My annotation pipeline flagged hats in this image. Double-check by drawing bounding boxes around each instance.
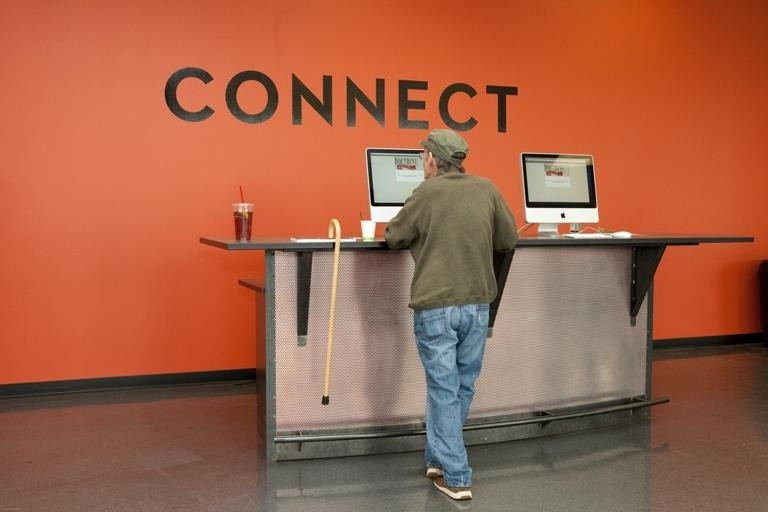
[418,128,470,165]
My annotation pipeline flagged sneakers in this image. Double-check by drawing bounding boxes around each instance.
[427,466,473,501]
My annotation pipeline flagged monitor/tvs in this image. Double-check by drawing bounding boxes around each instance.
[521,153,599,236]
[365,147,424,223]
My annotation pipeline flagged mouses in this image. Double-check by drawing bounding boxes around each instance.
[612,231,632,239]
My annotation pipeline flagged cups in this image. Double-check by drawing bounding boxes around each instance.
[359,219,376,242]
[231,202,255,242]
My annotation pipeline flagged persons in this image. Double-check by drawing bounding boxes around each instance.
[384,131,518,501]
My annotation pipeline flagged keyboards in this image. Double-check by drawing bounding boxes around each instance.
[563,233,613,239]
